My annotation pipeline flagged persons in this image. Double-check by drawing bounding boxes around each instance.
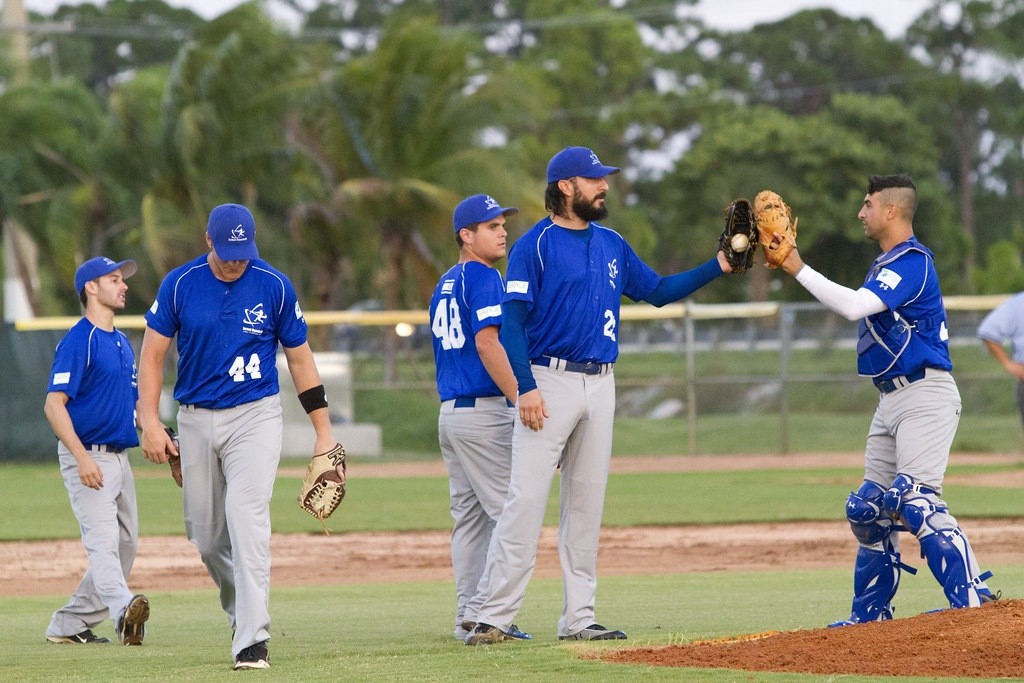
[755,174,993,628]
[428,194,531,642]
[45,257,150,646]
[138,204,347,671]
[466,146,758,646]
[977,293,1024,423]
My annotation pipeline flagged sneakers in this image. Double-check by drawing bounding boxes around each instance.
[115,594,152,647]
[464,622,505,646]
[828,616,886,627]
[462,619,531,640]
[47,629,112,644]
[558,625,628,641]
[234,643,270,670]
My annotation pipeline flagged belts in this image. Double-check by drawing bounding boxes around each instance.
[875,369,926,395]
[454,395,515,408]
[531,356,614,376]
[186,403,200,409]
[82,442,126,453]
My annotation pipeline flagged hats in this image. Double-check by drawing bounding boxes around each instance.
[207,203,260,261]
[547,146,622,185]
[75,256,137,297]
[452,193,518,233]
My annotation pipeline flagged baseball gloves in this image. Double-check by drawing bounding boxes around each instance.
[297,442,348,538]
[165,425,183,488]
[717,197,760,275]
[754,190,799,271]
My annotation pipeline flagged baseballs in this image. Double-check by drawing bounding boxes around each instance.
[730,233,750,253]
